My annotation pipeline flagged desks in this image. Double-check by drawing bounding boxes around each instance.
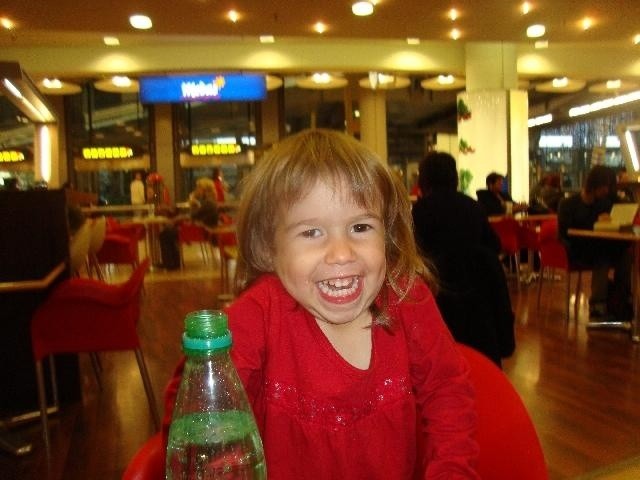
[567,226,640,342]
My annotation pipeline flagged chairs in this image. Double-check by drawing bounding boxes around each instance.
[489,215,584,305]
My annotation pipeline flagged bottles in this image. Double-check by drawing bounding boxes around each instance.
[165,309,269,480]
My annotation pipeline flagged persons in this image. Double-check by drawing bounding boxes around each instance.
[476,172,542,274]
[412,150,517,372]
[556,163,636,323]
[151,177,220,272]
[212,164,235,227]
[129,169,147,215]
[120,128,549,479]
